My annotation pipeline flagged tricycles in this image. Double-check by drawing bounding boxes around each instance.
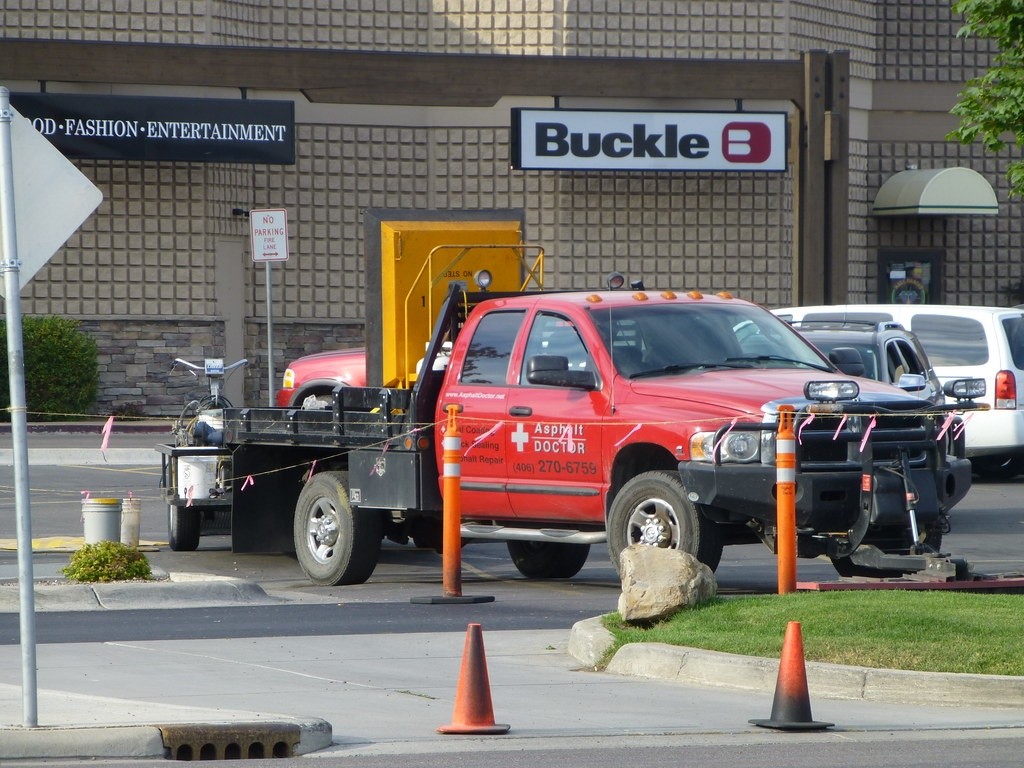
[155,356,250,551]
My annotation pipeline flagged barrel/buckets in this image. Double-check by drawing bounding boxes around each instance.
[80,499,123,544]
[175,447,218,499]
[121,499,141,547]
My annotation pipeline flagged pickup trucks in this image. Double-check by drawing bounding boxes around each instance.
[218,270,972,588]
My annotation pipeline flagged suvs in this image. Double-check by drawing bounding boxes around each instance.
[731,304,1024,481]
[731,318,948,411]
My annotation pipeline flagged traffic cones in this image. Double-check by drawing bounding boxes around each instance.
[747,619,837,732]
[435,624,514,735]
